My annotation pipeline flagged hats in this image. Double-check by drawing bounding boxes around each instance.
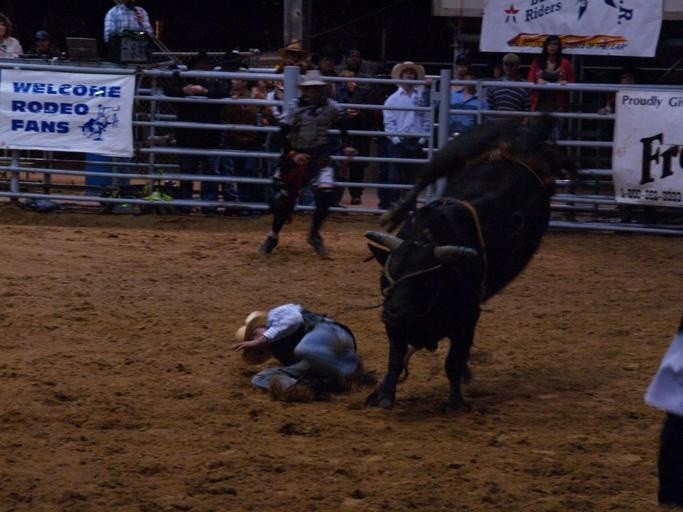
[234,310,273,366]
[34,30,53,40]
[454,53,472,64]
[216,49,250,69]
[295,68,330,89]
[278,38,313,62]
[189,52,218,69]
[502,53,521,66]
[311,47,344,65]
[392,59,426,88]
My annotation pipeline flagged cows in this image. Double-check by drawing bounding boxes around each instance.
[360,109,561,416]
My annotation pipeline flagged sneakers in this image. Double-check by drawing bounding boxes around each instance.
[202,206,223,216]
[223,207,271,217]
[350,195,362,205]
[306,231,329,259]
[256,231,279,254]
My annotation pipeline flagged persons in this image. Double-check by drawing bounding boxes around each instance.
[644,318,682,510]
[0,3,646,254]
[235,303,366,400]
